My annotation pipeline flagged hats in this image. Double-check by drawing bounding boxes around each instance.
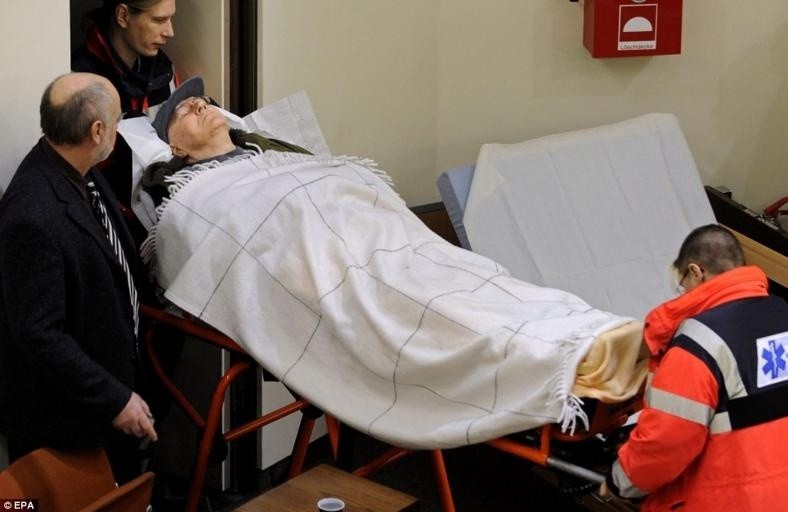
[152,74,205,144]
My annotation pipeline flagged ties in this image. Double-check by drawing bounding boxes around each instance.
[87,180,143,354]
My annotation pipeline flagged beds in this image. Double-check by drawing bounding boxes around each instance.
[105,122,651,504]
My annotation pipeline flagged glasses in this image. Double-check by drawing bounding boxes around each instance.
[170,94,212,125]
[675,268,706,296]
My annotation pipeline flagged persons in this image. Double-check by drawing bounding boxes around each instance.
[69,0,181,113]
[142,73,314,221]
[599,224,788,511]
[0,71,157,484]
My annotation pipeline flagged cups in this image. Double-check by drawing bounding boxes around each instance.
[316,496,346,512]
